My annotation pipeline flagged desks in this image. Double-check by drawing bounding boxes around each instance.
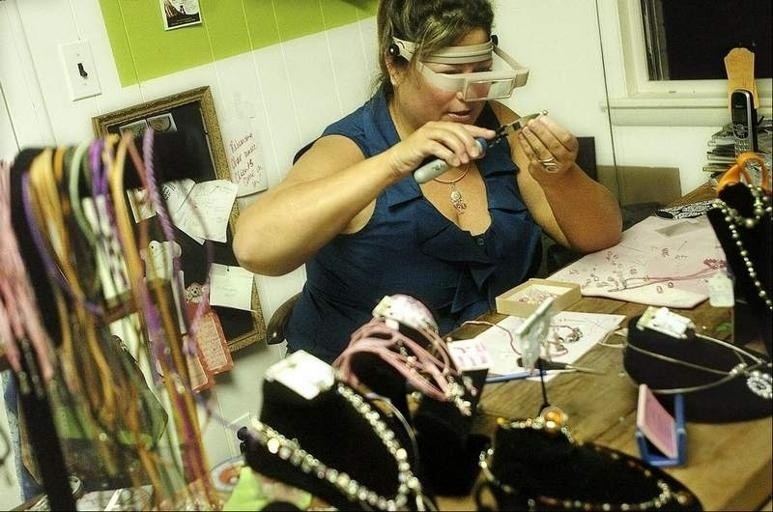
[141,186,773,512]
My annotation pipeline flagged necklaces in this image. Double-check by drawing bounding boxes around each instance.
[1,129,220,512]
[461,320,580,361]
[624,257,729,289]
[246,388,425,512]
[713,187,773,315]
[332,294,478,419]
[474,453,693,511]
[622,333,772,400]
[433,162,471,214]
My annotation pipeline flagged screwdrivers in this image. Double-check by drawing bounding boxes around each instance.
[518,357,608,376]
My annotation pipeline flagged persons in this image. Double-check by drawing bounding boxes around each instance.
[232,0,623,370]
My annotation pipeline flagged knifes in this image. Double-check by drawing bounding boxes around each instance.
[413,113,540,183]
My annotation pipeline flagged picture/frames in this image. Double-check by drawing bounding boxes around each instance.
[92,86,267,356]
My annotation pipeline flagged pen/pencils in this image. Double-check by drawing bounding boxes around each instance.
[485,370,546,383]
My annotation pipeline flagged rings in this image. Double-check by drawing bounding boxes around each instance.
[538,157,560,174]
